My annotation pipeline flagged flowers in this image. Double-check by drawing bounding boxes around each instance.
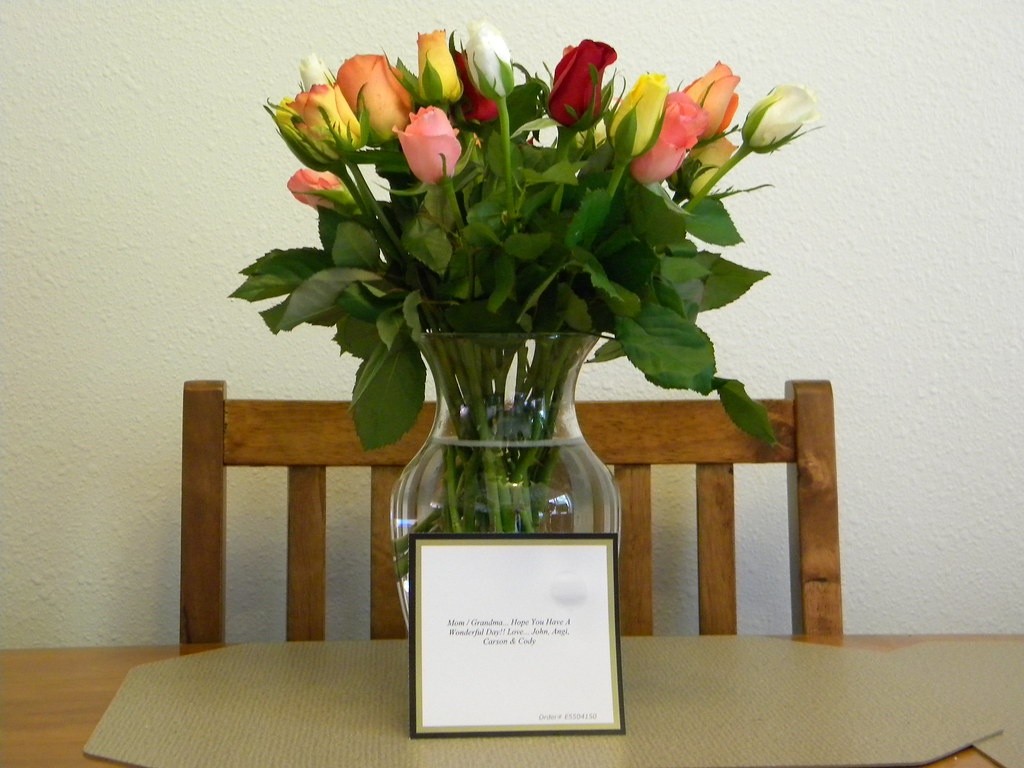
[229,26,825,452]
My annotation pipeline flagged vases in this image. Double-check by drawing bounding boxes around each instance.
[389,328,622,634]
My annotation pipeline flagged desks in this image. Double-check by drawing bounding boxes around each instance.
[0,635,1024,768]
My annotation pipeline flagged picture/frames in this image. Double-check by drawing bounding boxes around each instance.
[408,534,626,737]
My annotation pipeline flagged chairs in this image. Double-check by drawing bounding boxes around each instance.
[180,380,844,644]
